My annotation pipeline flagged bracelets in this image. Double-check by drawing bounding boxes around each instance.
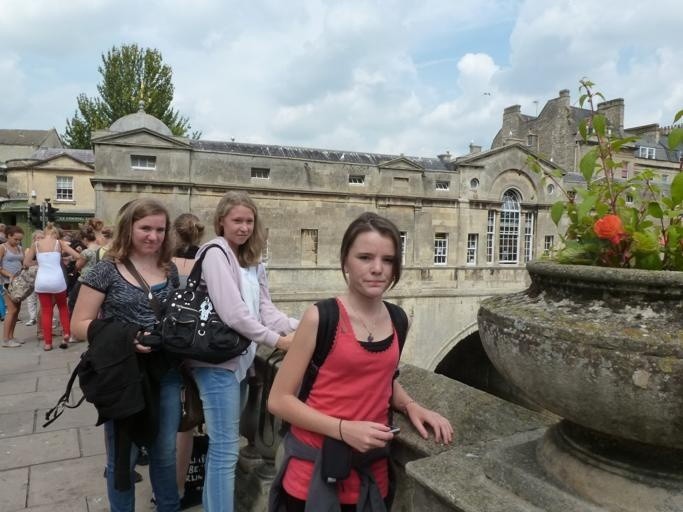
[401,398,414,416]
[339,418,344,442]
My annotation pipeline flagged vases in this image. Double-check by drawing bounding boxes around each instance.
[478,259,683,512]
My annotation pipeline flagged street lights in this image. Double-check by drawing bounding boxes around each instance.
[40,197,49,231]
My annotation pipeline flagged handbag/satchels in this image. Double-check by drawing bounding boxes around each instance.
[0,265,37,306]
[151,435,213,507]
[159,286,256,365]
[177,368,206,433]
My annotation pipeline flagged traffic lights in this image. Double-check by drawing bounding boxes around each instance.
[49,206,60,223]
[28,204,40,226]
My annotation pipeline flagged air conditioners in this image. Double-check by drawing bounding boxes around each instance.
[634,146,656,160]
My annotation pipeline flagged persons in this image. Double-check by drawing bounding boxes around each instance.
[150,212,205,509]
[69,197,181,512]
[186,191,301,512]
[104,449,150,482]
[265,213,454,512]
[0,217,116,351]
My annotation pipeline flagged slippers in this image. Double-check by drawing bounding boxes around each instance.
[58,336,70,350]
[42,344,53,352]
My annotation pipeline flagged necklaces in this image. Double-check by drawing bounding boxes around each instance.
[351,304,382,342]
[129,256,153,300]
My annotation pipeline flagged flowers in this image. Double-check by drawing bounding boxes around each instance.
[519,76,683,272]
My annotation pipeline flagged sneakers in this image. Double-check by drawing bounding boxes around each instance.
[0,338,25,349]
[23,317,36,327]
[149,483,203,510]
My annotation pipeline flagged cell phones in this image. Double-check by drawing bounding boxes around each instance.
[385,424,401,434]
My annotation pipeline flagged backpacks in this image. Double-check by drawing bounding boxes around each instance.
[275,292,405,437]
